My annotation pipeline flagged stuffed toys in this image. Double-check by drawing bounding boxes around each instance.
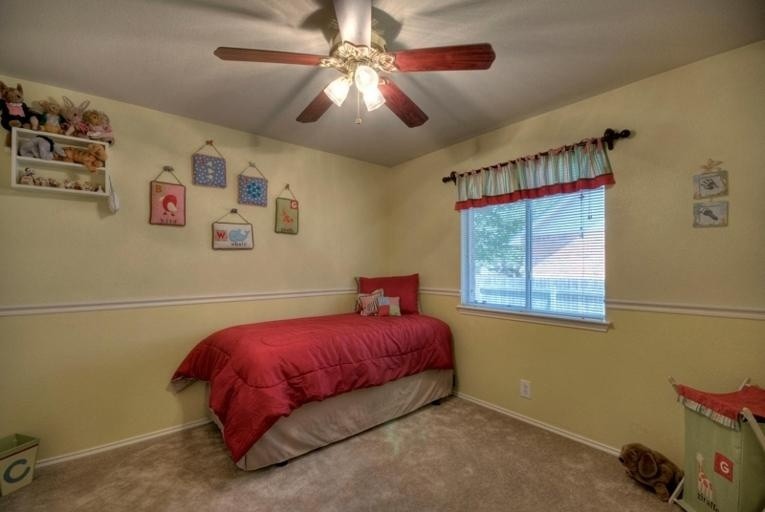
[0,81,41,130]
[19,135,66,161]
[619,443,684,501]
[84,111,114,145]
[37,100,65,134]
[52,144,106,174]
[62,96,91,137]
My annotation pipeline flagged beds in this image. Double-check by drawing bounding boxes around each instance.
[171,315,454,471]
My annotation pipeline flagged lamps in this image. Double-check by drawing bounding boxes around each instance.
[319,1,398,125]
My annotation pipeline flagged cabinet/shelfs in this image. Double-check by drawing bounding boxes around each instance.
[11,126,110,199]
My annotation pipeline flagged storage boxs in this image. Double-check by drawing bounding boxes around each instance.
[669,377,765,512]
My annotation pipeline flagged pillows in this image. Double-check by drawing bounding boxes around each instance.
[359,292,382,316]
[374,297,402,316]
[357,288,385,309]
[354,272,422,315]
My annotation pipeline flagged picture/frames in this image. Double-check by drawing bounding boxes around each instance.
[694,170,729,200]
[693,201,729,228]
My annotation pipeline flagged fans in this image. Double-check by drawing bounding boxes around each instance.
[213,1,496,129]
